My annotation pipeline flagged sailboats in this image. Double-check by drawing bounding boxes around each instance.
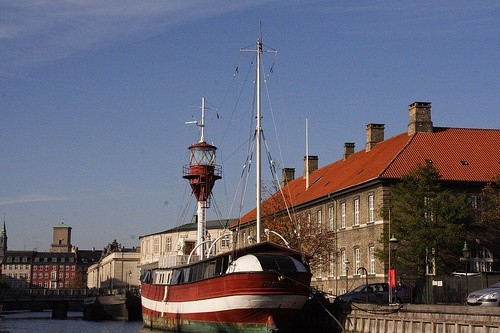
[139,17,314,333]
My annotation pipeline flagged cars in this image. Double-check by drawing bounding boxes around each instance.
[334,283,411,313]
[467,282,500,306]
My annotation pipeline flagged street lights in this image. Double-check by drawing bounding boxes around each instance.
[344,256,350,300]
[476,237,489,289]
[128,270,132,291]
[461,240,470,303]
[388,233,400,303]
